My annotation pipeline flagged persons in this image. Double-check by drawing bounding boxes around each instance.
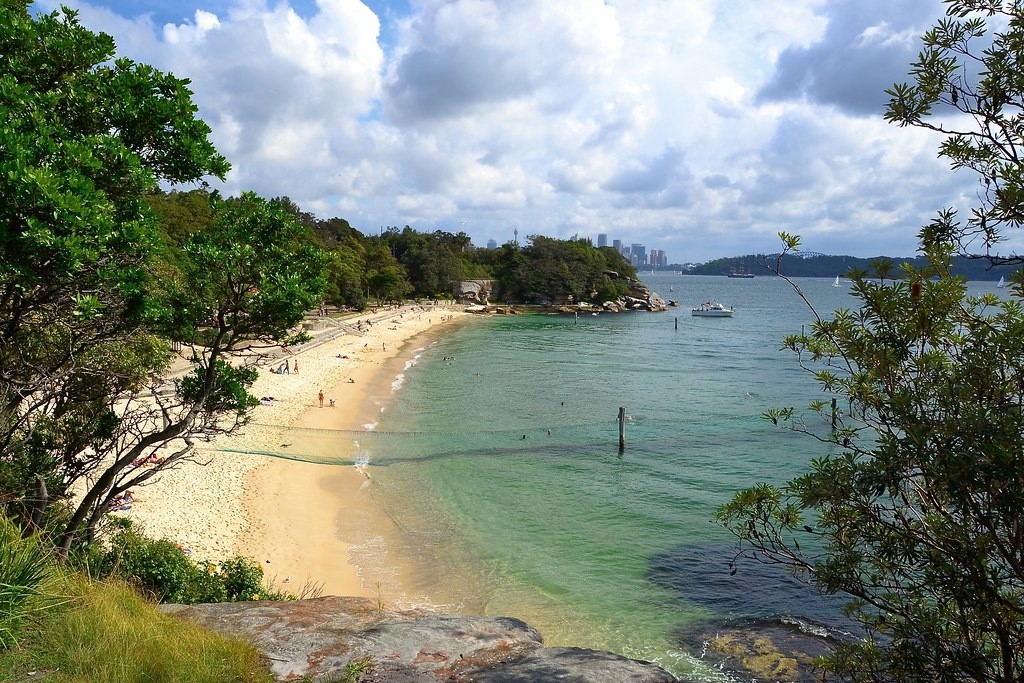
[132,452,168,468]
[319,389,338,407]
[318,303,453,331]
[477,373,479,376]
[282,347,299,375]
[522,401,564,439]
[443,356,455,361]
[338,342,385,358]
[109,490,139,507]
[348,377,355,383]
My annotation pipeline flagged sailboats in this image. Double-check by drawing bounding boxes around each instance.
[728,254,756,278]
[831,274,841,288]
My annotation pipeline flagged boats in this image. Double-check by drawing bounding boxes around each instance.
[692,301,734,317]
